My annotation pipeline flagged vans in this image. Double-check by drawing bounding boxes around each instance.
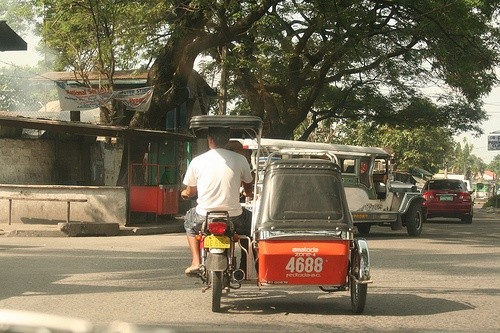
[227,138,424,236]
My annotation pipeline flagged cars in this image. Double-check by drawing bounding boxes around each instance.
[420,178,475,225]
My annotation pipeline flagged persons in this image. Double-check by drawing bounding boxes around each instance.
[181,128,253,275]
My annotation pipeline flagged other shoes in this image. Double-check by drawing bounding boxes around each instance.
[185,265,200,273]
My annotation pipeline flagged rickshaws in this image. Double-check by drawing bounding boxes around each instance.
[182,115,374,313]
